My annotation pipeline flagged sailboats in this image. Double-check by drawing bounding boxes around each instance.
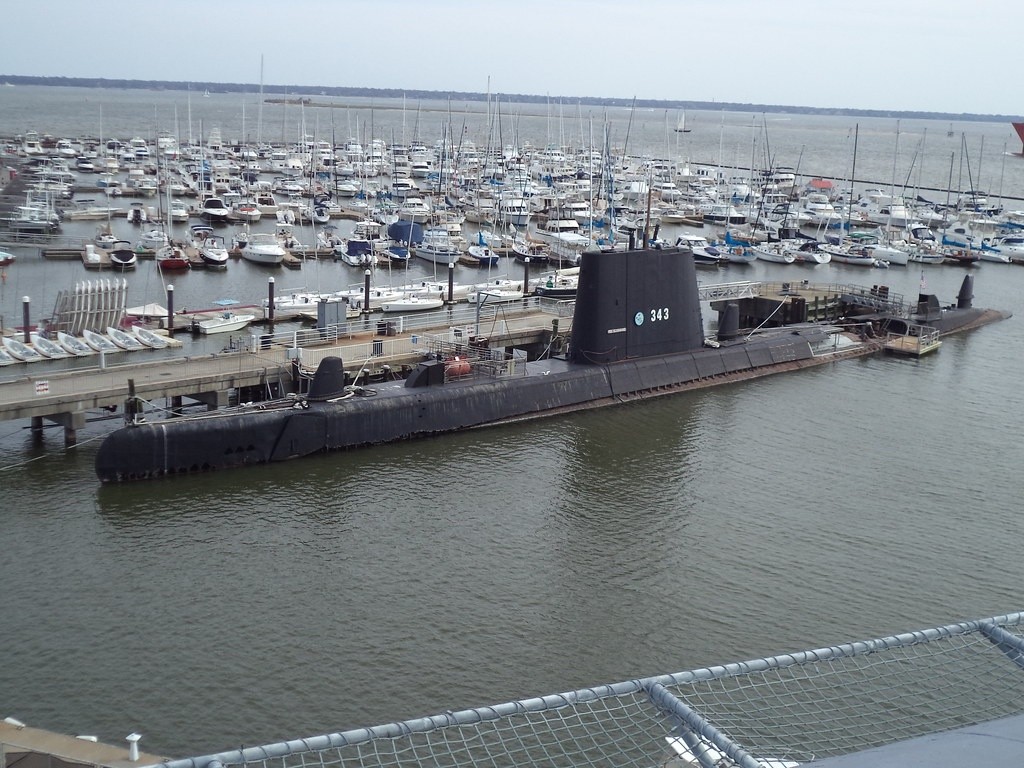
[0,74,1024,324]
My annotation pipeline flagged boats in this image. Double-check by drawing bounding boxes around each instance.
[0,349,14,366]
[30,334,68,359]
[83,330,118,354]
[108,327,142,350]
[187,306,256,335]
[58,332,93,356]
[132,325,168,349]
[1,336,42,363]
